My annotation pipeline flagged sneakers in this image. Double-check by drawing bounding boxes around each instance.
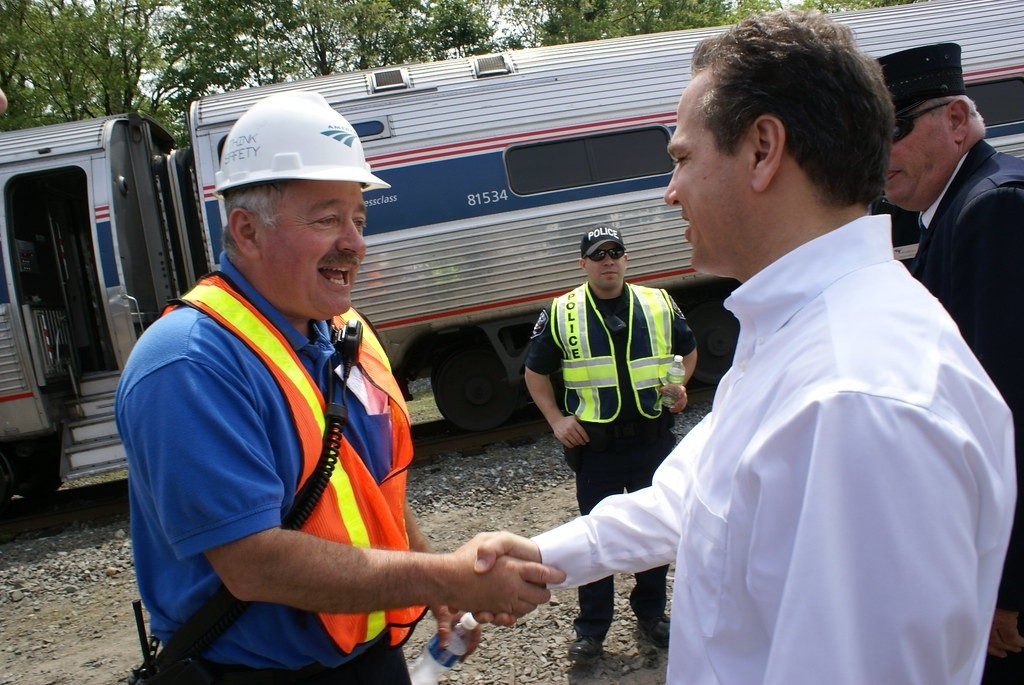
[636,614,670,647]
[568,635,604,665]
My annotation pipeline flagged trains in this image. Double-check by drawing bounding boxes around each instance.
[0,1,1024,524]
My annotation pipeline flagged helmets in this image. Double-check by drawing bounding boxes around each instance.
[214,93,393,192]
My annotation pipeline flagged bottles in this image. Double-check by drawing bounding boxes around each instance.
[664,355,685,407]
[409,612,479,685]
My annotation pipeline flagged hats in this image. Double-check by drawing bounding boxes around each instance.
[873,43,967,116]
[581,224,626,259]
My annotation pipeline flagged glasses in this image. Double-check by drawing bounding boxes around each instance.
[891,100,950,144]
[588,246,624,261]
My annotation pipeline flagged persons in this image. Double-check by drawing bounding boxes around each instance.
[876,41,1024,685]
[521,223,699,665]
[448,8,1017,685]
[114,92,566,685]
